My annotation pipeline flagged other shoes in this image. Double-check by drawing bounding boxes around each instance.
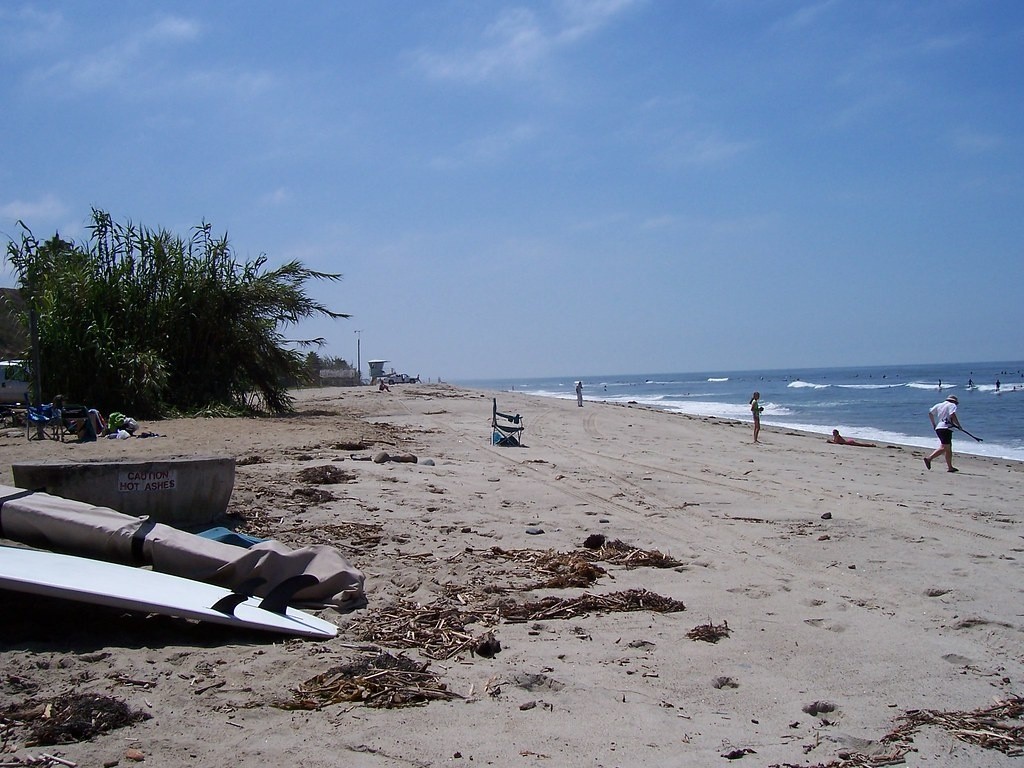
[947,467,958,472]
[924,458,932,470]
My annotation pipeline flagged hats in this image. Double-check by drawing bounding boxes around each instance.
[945,395,958,404]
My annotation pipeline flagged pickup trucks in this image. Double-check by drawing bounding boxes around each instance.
[383,373,416,385]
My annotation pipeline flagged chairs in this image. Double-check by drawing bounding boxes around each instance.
[492,398,524,448]
[24,391,63,441]
[49,406,97,442]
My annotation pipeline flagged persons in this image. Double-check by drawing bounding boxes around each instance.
[939,379,941,386]
[750,392,764,443]
[968,379,973,388]
[923,395,962,472]
[576,382,583,407]
[379,380,391,392]
[826,430,876,447]
[995,379,1000,392]
[51,395,75,433]
[417,375,421,383]
